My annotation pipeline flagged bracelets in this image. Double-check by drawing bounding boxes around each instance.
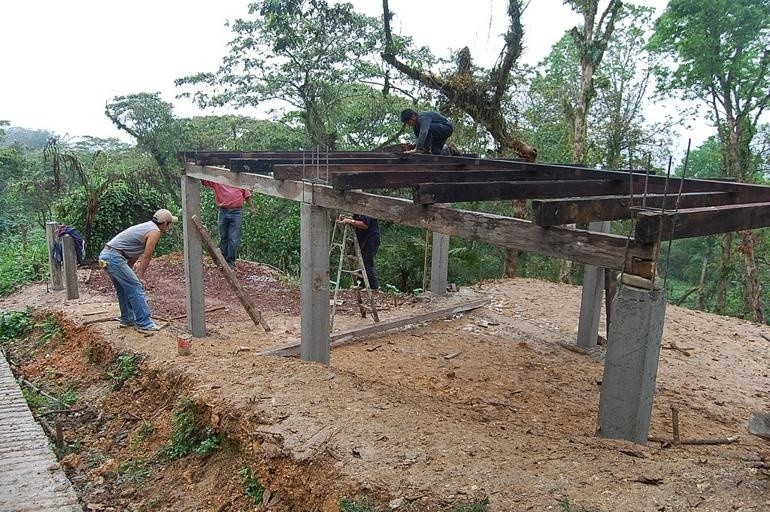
[351,219,356,226]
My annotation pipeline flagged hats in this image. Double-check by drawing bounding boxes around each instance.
[153,209,179,224]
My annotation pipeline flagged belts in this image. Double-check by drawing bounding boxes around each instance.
[104,244,126,256]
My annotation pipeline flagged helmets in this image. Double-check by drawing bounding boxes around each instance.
[401,109,413,122]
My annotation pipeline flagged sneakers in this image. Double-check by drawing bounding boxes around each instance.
[120,322,134,328]
[134,325,161,331]
[449,142,463,157]
[353,286,379,292]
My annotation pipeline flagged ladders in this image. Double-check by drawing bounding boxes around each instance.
[191,213,272,332]
[329,215,380,333]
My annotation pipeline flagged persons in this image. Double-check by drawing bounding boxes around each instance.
[399,109,465,156]
[335,187,382,292]
[97,207,180,332]
[201,180,257,265]
